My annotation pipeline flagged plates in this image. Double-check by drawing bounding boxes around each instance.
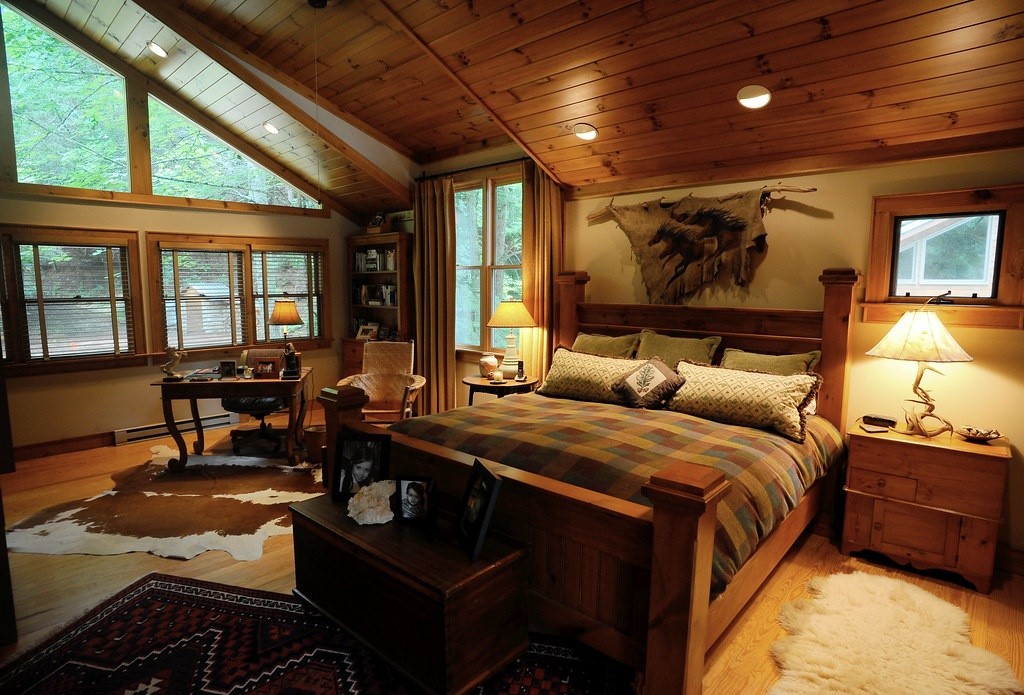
[490,380,507,383]
[955,431,1002,441]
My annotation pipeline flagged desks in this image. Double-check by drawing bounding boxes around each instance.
[461,374,539,407]
[149,366,314,466]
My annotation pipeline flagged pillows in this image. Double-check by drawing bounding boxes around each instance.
[610,355,686,411]
[634,329,722,371]
[719,347,822,377]
[569,331,641,358]
[534,343,650,404]
[664,358,824,443]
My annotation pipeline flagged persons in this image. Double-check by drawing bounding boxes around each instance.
[339,447,373,492]
[401,482,426,518]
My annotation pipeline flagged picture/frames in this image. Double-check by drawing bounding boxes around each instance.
[450,457,502,564]
[394,475,435,523]
[355,325,380,339]
[331,431,390,505]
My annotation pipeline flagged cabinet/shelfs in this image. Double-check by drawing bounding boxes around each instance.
[344,232,412,375]
[840,419,1013,594]
[287,494,530,695]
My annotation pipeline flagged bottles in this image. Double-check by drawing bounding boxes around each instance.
[479,352,500,376]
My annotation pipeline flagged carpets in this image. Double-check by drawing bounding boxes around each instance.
[4,421,331,561]
[0,573,646,694]
[767,570,1024,695]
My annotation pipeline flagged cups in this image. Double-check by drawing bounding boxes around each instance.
[493,370,504,381]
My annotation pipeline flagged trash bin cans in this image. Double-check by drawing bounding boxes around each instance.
[303,425,327,463]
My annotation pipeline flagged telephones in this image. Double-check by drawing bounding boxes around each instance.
[515,361,527,382]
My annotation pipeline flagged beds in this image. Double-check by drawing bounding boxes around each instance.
[314,267,864,695]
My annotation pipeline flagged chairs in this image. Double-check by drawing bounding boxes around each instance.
[220,349,305,453]
[337,339,427,430]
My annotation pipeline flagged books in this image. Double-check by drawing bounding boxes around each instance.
[352,316,370,336]
[351,280,396,308]
[355,248,397,271]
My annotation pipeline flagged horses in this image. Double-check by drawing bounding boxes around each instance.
[160,347,188,376]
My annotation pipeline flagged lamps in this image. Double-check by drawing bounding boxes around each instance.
[267,300,305,372]
[865,300,974,437]
[486,301,539,378]
